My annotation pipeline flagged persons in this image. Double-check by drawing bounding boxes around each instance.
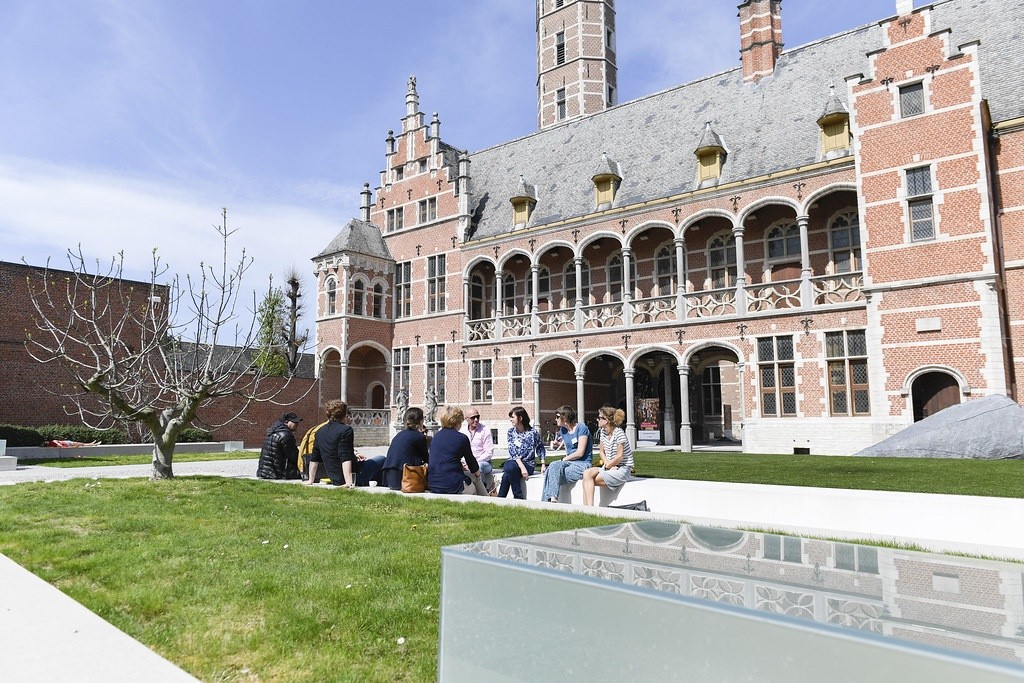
[297,400,385,488]
[640,417,659,430]
[497,406,546,500]
[547,431,564,451]
[41,440,101,446]
[428,405,496,496]
[420,425,432,447]
[540,405,593,503]
[381,407,429,490]
[256,412,302,479]
[582,406,635,505]
[587,419,596,436]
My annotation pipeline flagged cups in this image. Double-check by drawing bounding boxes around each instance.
[349,473,356,489]
[369,481,378,487]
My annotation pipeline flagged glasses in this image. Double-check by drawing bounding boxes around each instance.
[468,415,480,420]
[597,416,607,420]
[556,415,561,419]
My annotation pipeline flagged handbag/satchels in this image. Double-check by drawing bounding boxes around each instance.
[401,463,428,493]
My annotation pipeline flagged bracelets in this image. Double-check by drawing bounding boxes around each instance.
[541,463,545,464]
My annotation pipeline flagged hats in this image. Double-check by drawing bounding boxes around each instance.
[280,412,304,422]
